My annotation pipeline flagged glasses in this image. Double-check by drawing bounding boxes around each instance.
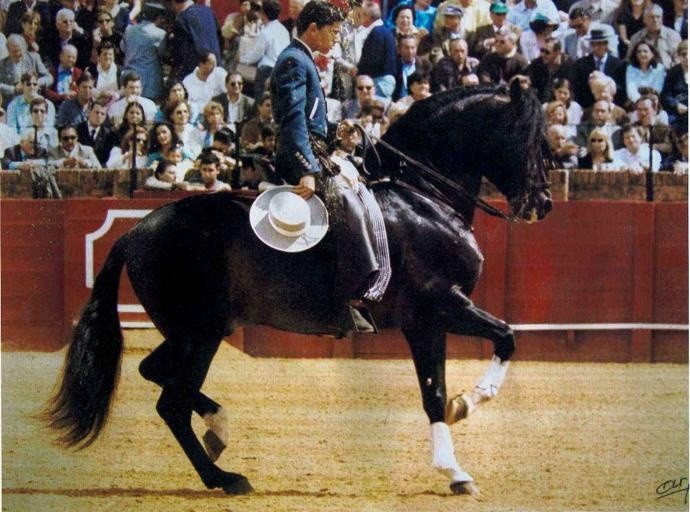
[230,80,243,88]
[539,47,552,56]
[358,85,374,92]
[61,135,78,143]
[591,138,606,143]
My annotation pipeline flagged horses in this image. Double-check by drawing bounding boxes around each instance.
[20,74,556,497]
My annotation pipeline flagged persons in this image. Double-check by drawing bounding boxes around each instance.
[0,0,688,193]
[271,0,394,339]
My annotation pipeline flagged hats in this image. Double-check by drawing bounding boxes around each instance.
[583,25,619,44]
[248,182,329,253]
[441,5,464,18]
[489,1,509,15]
